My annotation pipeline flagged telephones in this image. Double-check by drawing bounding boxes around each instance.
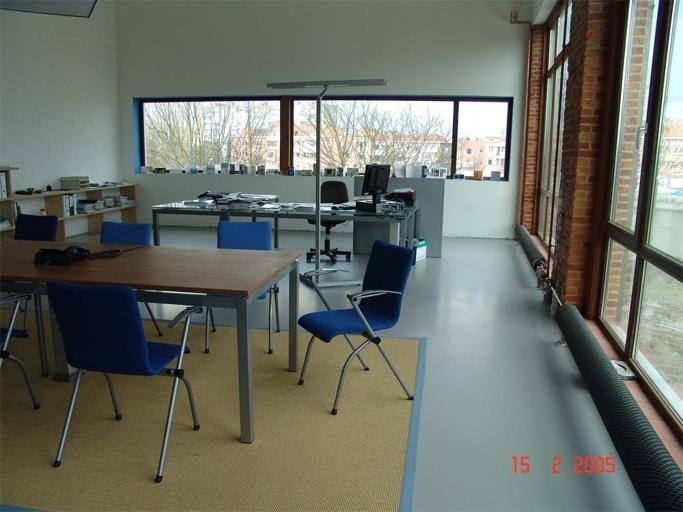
[35,245,122,265]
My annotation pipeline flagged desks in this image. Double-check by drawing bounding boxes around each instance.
[0,239,304,445]
[152,198,419,249]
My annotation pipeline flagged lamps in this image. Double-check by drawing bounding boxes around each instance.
[266,78,388,287]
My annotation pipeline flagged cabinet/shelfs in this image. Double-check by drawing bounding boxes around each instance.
[0,183,140,240]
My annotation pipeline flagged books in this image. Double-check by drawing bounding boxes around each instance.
[65,194,77,216]
[78,199,104,214]
[258,202,282,212]
[104,195,129,208]
[218,196,258,212]
[314,202,356,217]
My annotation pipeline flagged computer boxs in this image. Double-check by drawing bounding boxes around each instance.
[357,199,401,214]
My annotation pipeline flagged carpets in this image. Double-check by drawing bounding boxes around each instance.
[0,306,427,512]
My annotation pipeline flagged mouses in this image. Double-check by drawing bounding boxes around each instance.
[330,205,340,211]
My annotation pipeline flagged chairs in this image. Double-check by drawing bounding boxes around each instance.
[305,181,351,263]
[297,238,415,416]
[205,221,280,355]
[0,293,41,410]
[46,281,204,482]
[14,213,59,329]
[99,221,163,337]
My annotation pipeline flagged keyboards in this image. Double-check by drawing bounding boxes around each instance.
[339,205,356,210]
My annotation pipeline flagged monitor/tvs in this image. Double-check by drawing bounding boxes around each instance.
[360,163,388,205]
